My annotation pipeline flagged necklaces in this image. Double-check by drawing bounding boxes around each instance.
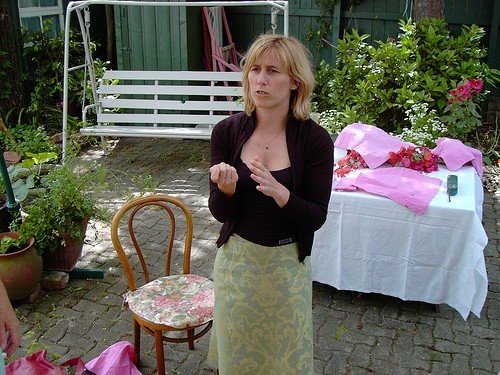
[260,127,285,149]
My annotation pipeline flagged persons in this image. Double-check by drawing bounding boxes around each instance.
[205,34,335,375]
[0,279,21,375]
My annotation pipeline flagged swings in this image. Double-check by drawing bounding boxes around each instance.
[78,5,279,139]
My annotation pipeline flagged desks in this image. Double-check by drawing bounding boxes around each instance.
[311,166,489,321]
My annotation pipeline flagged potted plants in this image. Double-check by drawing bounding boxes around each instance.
[0,122,166,306]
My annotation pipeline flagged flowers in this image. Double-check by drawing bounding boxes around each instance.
[387,103,449,172]
[334,147,368,176]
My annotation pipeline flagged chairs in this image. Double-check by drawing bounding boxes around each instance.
[110,194,221,375]
